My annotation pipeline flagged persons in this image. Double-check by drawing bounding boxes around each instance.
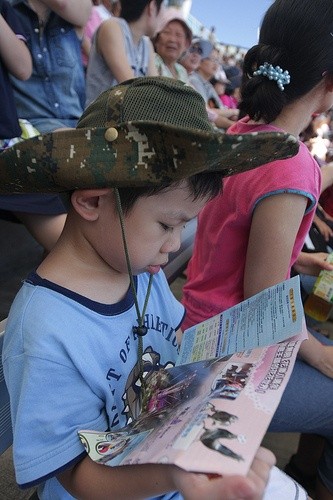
[175,0,333,435]
[0,76,312,500]
[0,0,333,322]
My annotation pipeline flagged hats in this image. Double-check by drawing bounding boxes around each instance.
[0,75,300,191]
[151,7,193,50]
[191,34,214,60]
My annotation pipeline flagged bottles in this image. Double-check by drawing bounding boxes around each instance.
[304,253,333,323]
[308,112,330,164]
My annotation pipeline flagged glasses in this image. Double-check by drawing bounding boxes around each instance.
[189,46,205,54]
